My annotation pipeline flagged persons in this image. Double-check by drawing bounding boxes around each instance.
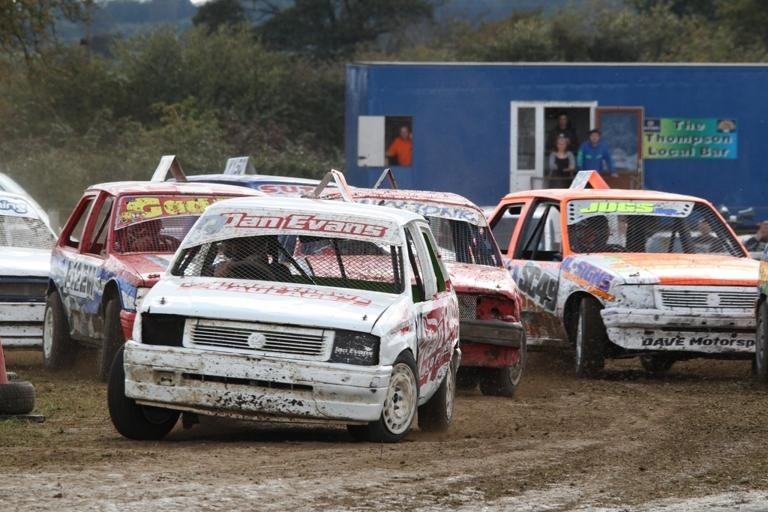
[547,132,575,175]
[386,121,413,166]
[743,217,767,252]
[694,213,726,253]
[574,128,620,178]
[546,112,576,154]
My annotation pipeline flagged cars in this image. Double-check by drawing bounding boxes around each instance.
[108,169,466,442]
[43,154,271,385]
[0,174,57,368]
[468,168,761,378]
[169,155,362,195]
[645,229,731,255]
[476,203,564,260]
[304,170,532,396]
[753,219,767,376]
